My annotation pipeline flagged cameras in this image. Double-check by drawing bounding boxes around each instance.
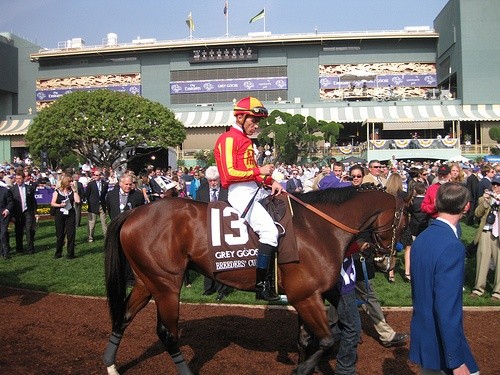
[347,176,353,181]
[489,191,496,198]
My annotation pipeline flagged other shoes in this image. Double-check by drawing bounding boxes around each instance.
[66,253,72,259]
[489,296,500,301]
[470,293,483,299]
[89,239,93,242]
[382,333,407,346]
[404,274,412,283]
[54,254,62,259]
[389,272,396,283]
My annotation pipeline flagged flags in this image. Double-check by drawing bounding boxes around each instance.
[224,2,227,15]
[249,9,264,24]
[186,15,194,30]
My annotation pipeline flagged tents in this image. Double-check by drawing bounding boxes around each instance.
[338,69,379,98]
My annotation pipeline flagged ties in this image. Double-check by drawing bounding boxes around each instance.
[18,187,24,209]
[492,196,500,238]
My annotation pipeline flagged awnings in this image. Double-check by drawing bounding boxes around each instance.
[0,105,500,135]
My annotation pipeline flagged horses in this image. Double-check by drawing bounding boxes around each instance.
[97,181,419,375]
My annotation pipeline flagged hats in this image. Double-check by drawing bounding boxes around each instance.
[438,164,451,175]
[490,174,500,183]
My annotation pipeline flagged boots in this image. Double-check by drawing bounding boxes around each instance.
[254,267,281,301]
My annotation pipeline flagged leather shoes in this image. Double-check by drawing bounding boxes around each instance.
[203,287,217,296]
[217,292,232,301]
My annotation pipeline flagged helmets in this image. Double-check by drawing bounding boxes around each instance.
[233,97,269,135]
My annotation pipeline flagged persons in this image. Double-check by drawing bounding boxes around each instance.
[324,140,360,148]
[347,82,457,100]
[370,131,376,140]
[421,160,500,300]
[436,131,453,138]
[409,182,480,375]
[214,96,282,302]
[465,139,471,145]
[275,160,429,375]
[0,155,228,300]
[257,143,274,165]
[413,132,420,139]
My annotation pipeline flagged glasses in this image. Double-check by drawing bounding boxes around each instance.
[233,106,269,115]
[93,174,98,176]
[370,166,381,169]
[491,182,500,185]
[351,175,362,178]
[293,173,298,175]
[422,172,428,176]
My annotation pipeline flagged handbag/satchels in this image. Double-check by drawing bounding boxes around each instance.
[49,206,56,216]
